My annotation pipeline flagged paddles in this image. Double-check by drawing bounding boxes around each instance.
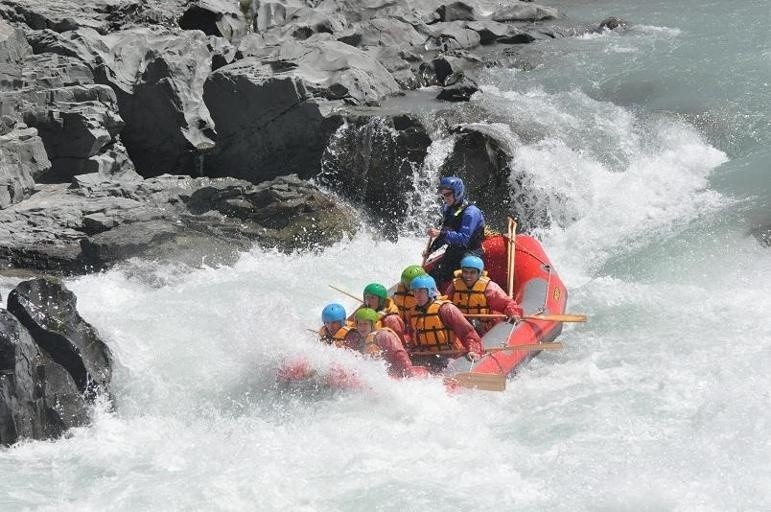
[444,372,506,392]
[463,313,587,323]
[410,342,564,356]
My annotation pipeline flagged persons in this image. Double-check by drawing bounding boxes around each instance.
[421,177,485,287]
[354,307,412,378]
[390,265,426,322]
[318,304,363,354]
[361,283,405,340]
[407,275,482,372]
[447,254,521,338]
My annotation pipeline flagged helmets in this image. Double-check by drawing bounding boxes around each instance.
[401,265,427,283]
[409,274,436,300]
[363,283,388,307]
[321,304,347,328]
[459,255,485,274]
[436,176,465,206]
[353,308,378,329]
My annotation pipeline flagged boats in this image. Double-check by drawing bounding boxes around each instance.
[277,233,569,398]
